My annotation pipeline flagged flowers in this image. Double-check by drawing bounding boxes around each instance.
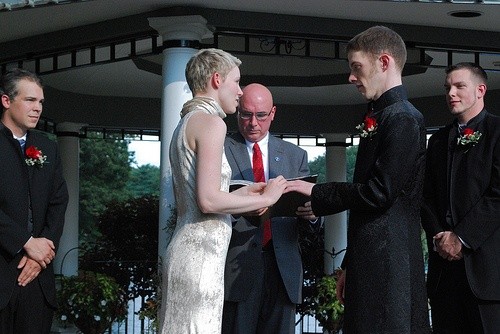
[55,270,129,334]
[354,117,379,142]
[456,127,482,151]
[23,145,52,170]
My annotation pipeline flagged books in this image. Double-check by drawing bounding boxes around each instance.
[230,173,318,217]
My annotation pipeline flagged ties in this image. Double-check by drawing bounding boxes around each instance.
[251,143,272,249]
[19,138,26,153]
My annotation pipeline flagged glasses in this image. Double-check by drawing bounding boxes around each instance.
[238,107,273,121]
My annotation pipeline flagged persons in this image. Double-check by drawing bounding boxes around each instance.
[0,67,69,334]
[283,25,434,334]
[224,82,324,334]
[420,62,500,334]
[158,48,287,334]
